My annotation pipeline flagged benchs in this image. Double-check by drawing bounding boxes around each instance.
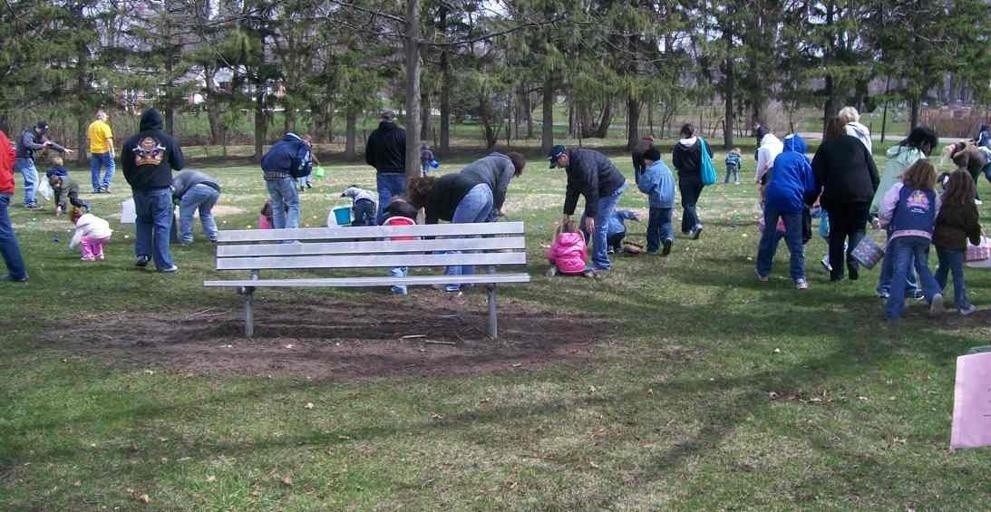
[203,221,530,340]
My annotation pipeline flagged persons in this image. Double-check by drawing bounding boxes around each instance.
[606,206,643,254]
[257,129,321,245]
[545,220,594,279]
[547,144,628,272]
[632,133,656,185]
[120,107,184,272]
[171,169,223,245]
[723,146,741,184]
[336,109,527,294]
[638,149,676,255]
[672,123,712,240]
[752,106,880,289]
[0,104,118,281]
[870,123,991,321]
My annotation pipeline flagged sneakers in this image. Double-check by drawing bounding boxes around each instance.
[3,201,177,282]
[755,258,975,317]
[545,223,703,279]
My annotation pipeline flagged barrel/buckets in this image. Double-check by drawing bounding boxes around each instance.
[332,198,351,225]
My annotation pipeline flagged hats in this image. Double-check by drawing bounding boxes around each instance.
[546,145,565,168]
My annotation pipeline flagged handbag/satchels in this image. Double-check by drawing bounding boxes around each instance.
[965,230,989,260]
[701,154,715,186]
